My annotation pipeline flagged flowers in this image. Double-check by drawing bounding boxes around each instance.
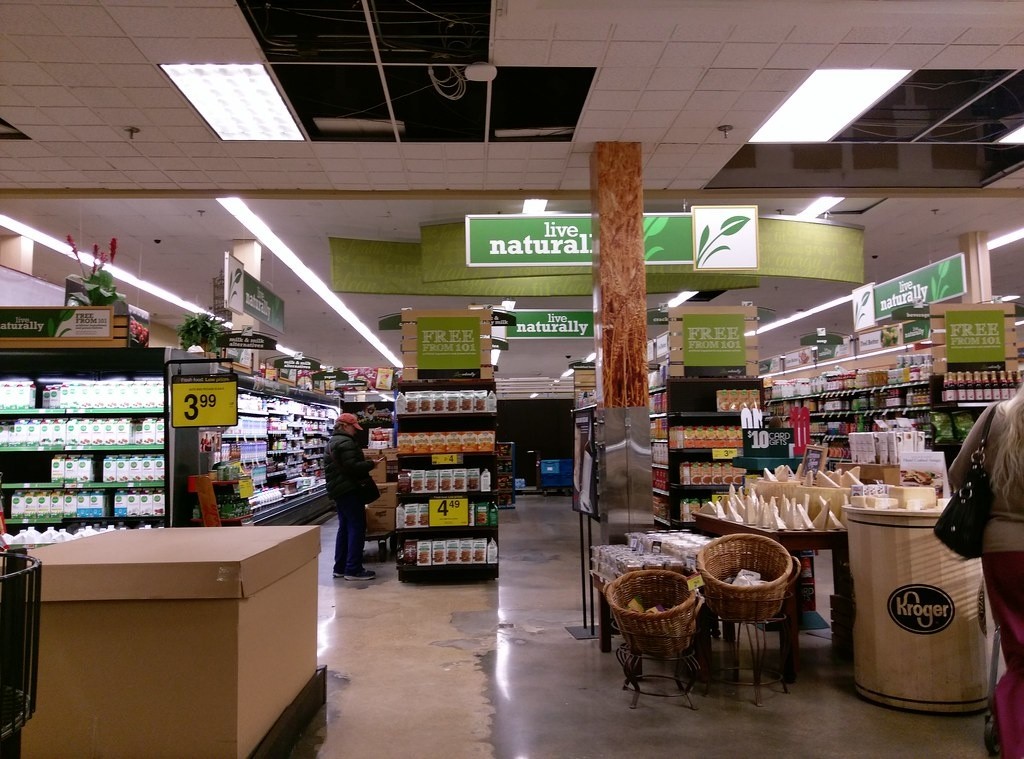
[65,232,127,308]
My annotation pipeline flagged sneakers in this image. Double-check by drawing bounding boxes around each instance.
[344,569,376,581]
[333,569,344,578]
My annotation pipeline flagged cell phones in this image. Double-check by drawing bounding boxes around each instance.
[379,457,385,460]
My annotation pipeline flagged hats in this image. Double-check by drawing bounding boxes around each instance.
[335,413,363,431]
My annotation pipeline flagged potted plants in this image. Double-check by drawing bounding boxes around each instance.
[178,310,220,353]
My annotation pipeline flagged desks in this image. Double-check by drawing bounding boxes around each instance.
[691,509,848,683]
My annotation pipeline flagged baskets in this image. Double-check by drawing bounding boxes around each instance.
[603,568,705,659]
[690,533,801,622]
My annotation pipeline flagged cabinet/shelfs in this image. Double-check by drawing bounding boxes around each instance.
[574,302,1024,526]
[393,379,500,582]
[496,441,516,509]
[188,473,254,527]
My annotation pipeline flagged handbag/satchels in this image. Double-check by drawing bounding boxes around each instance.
[933,402,998,559]
[348,473,381,505]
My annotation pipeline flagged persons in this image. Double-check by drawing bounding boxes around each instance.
[324,414,380,580]
[948,384,1024,759]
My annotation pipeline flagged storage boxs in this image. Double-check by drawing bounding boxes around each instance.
[0,525,321,759]
[742,428,798,458]
[361,430,496,534]
[376,367,393,391]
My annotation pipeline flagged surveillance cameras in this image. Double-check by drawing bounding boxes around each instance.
[566,355,571,358]
[872,255,878,259]
[154,239,161,243]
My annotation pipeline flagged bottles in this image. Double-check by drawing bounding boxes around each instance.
[11,521,166,545]
[480,468,492,491]
[766,360,1024,446]
